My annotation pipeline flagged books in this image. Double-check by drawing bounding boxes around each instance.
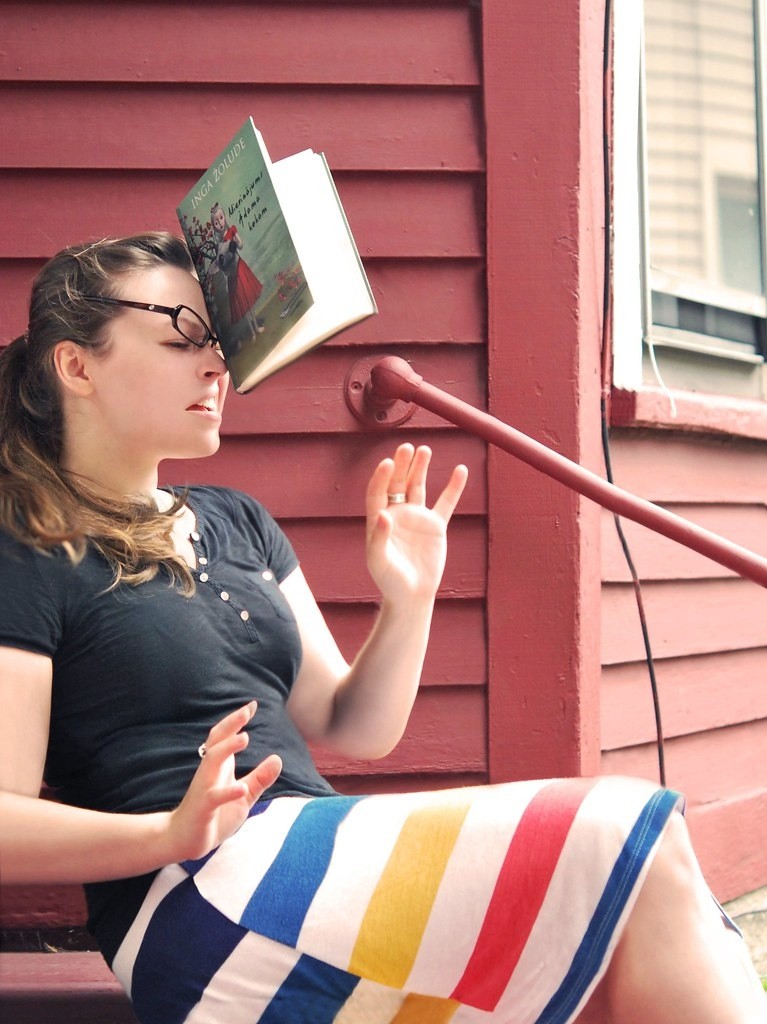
[177,114,379,396]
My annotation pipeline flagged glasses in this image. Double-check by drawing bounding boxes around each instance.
[81,295,220,348]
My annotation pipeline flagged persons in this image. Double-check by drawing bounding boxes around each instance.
[0,230,767,1024]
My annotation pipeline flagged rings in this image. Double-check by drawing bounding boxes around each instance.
[389,492,405,503]
[198,744,207,758]
